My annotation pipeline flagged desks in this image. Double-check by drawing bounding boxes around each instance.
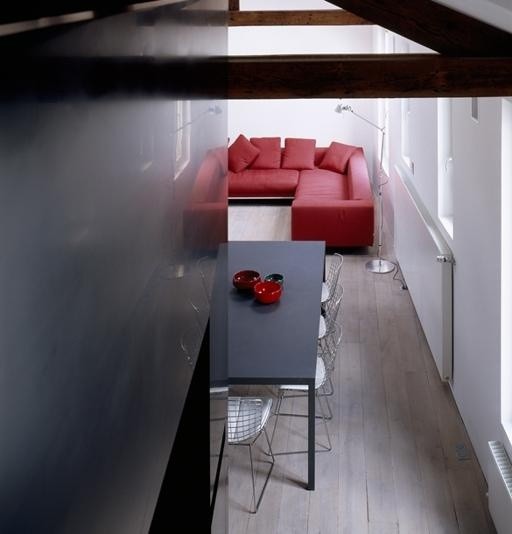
[228,241,326,490]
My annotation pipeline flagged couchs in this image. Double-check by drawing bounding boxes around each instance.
[183,147,228,250]
[228,146,374,248]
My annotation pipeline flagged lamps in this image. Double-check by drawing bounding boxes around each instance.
[160,104,223,280]
[334,103,395,274]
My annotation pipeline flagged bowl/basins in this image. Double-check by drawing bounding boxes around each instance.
[265,273,284,283]
[254,280,283,304]
[233,270,260,291]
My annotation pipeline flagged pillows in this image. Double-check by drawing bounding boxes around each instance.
[212,146,228,177]
[282,138,316,170]
[318,142,356,175]
[249,137,281,169]
[228,134,260,173]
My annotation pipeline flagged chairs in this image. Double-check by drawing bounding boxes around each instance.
[180,256,228,370]
[266,253,343,457]
[228,396,275,513]
